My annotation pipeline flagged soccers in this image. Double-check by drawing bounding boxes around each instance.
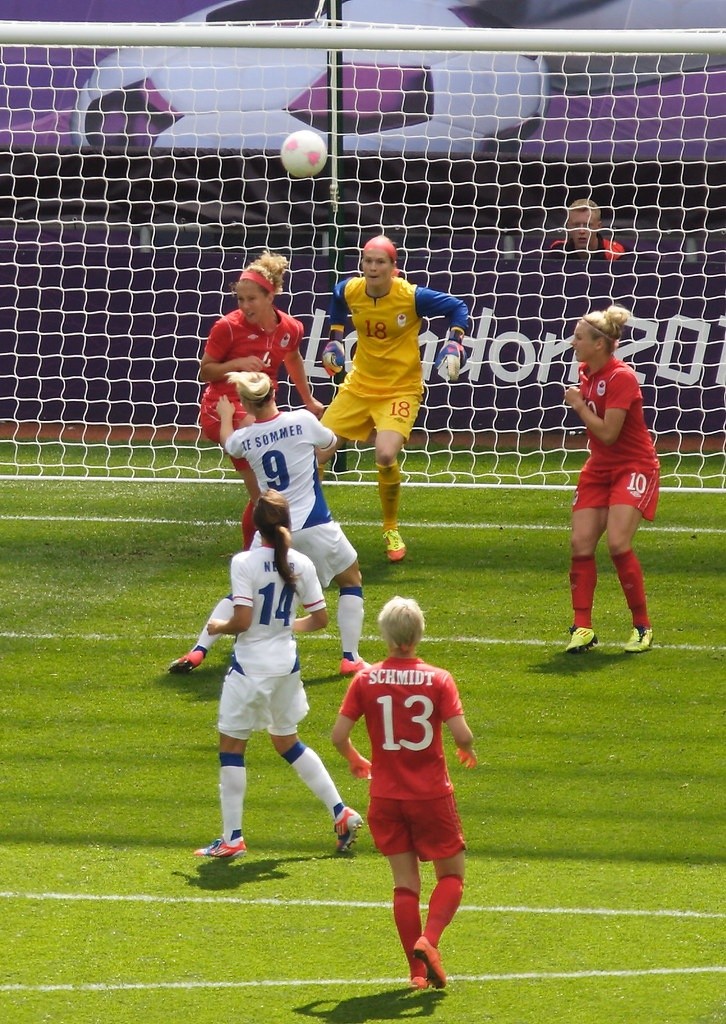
[281,130,328,178]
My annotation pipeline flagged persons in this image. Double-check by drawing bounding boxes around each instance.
[199,254,326,555]
[315,235,470,563]
[167,370,376,678]
[563,303,662,656]
[192,488,365,859]
[331,596,478,994]
[545,197,635,261]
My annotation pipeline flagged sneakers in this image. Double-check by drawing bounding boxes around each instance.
[566,625,598,653]
[334,807,364,852]
[383,530,407,563]
[194,837,247,858]
[341,658,373,675]
[412,977,428,990]
[168,651,203,674]
[624,626,654,653]
[414,936,446,989]
